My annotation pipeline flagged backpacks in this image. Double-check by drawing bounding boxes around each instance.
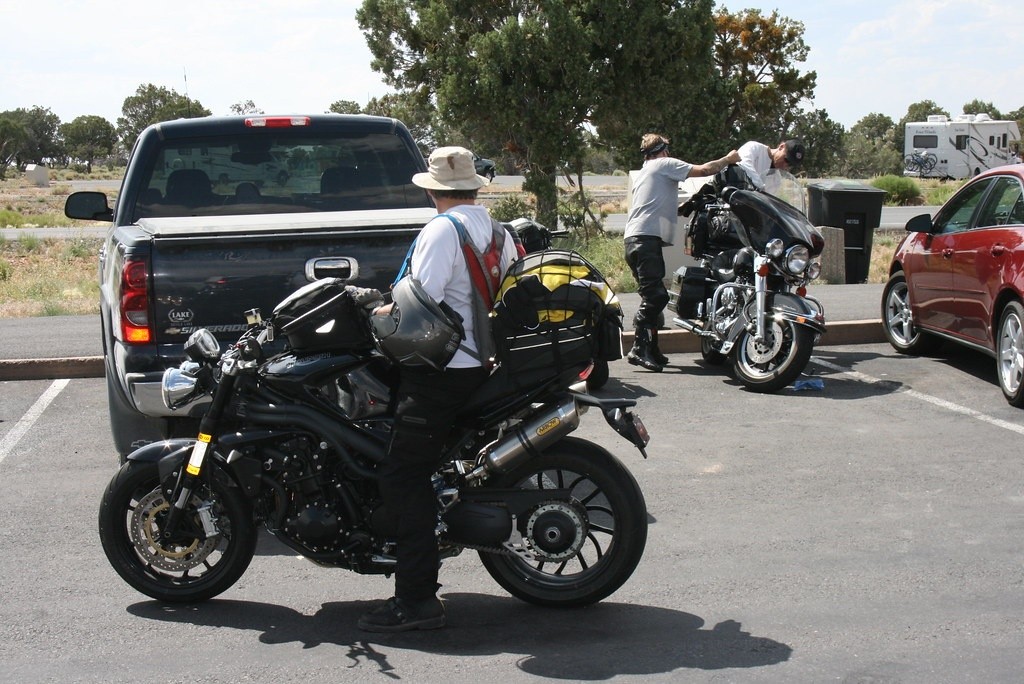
[487,248,624,366]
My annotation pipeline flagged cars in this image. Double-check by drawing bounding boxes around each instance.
[470,151,496,184]
[881,163,1024,410]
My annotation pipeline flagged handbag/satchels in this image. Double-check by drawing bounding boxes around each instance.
[269,276,380,358]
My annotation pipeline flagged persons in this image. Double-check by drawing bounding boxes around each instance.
[736,140,805,193]
[356,145,514,632]
[626,134,742,372]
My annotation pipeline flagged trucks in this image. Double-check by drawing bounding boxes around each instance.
[903,113,1023,179]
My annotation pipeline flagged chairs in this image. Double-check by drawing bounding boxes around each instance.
[165,170,213,204]
[320,165,361,197]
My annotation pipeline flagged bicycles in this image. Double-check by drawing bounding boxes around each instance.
[904,149,938,175]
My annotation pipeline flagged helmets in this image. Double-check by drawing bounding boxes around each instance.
[374,275,465,374]
[713,162,750,199]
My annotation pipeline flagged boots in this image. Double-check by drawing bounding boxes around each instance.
[648,328,669,364]
[626,326,663,372]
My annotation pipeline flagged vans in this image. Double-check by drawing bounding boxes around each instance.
[162,145,288,187]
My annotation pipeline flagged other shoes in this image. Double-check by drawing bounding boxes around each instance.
[356,596,447,632]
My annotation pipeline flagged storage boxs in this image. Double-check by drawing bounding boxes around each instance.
[666,264,710,320]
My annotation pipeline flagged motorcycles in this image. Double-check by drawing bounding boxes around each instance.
[508,217,612,391]
[98,274,657,614]
[666,162,828,394]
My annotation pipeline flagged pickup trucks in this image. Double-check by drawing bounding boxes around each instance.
[64,112,443,471]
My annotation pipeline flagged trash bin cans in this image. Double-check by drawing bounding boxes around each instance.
[806,178,888,284]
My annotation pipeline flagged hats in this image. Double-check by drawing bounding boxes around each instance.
[785,139,805,165]
[412,146,490,190]
